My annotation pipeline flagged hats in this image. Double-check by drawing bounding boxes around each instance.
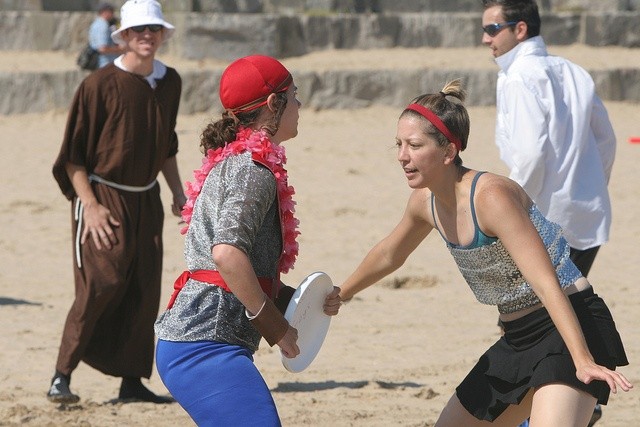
[111,0,174,48]
[219,54,292,115]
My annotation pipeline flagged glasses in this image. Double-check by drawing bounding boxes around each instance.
[129,25,163,31]
[481,23,517,33]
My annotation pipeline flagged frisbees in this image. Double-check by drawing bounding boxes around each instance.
[282,272,335,373]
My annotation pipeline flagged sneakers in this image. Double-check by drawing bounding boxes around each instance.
[119,385,174,404]
[48,381,80,405]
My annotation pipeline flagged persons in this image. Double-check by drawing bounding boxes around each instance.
[337,77,635,427]
[47,0,188,407]
[477,1,621,282]
[88,2,115,71]
[151,54,341,427]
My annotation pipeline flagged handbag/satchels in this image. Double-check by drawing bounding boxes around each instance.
[77,47,99,70]
